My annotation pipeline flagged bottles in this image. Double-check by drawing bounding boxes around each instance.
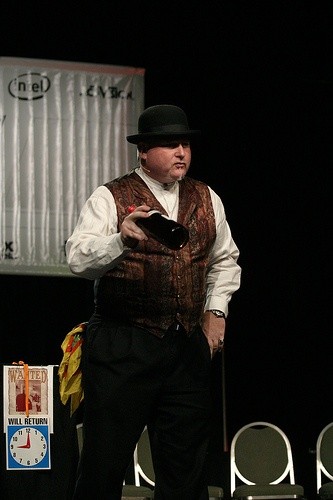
[127,205,189,250]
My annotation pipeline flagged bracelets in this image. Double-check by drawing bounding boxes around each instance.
[206,310,226,319]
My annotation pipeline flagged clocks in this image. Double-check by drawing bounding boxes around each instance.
[6,415,51,470]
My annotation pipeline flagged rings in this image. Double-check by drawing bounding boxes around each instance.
[219,337,224,345]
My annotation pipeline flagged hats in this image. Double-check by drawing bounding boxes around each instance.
[126,104,201,144]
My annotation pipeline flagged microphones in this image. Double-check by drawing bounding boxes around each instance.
[140,165,150,174]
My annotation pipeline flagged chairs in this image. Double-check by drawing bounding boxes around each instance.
[75,421,223,500]
[316,423,333,500]
[230,422,304,500]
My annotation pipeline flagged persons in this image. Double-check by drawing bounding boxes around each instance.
[65,105,242,500]
